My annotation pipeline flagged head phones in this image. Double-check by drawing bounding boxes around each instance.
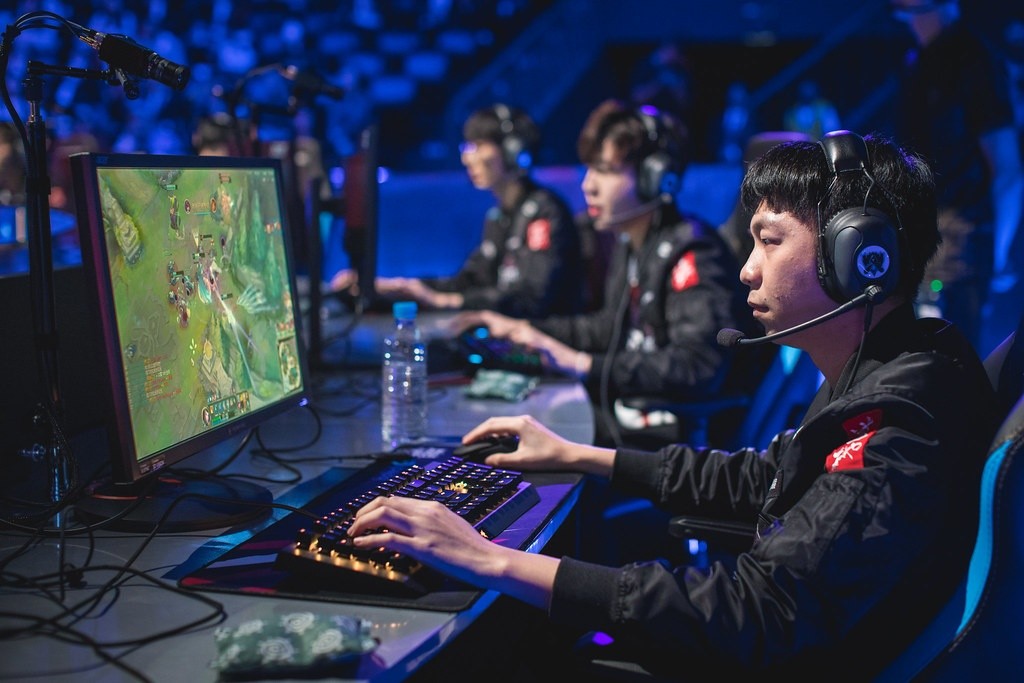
[494,102,530,170]
[815,129,905,308]
[638,105,681,204]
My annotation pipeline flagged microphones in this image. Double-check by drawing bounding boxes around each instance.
[81,29,190,92]
[608,194,671,227]
[717,286,885,348]
[112,64,141,99]
[278,64,344,102]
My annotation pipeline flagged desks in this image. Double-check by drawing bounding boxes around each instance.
[1,310,595,683]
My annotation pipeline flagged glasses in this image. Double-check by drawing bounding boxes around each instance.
[459,141,491,154]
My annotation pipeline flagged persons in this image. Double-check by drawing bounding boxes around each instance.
[194,113,255,160]
[0,121,93,214]
[439,102,754,429]
[329,106,579,319]
[348,125,997,683]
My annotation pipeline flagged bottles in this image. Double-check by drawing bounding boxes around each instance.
[380,302,428,444]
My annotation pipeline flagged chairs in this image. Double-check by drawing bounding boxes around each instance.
[856,403,1024,682]
[603,337,822,581]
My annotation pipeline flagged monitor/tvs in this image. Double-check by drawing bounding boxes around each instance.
[68,126,379,534]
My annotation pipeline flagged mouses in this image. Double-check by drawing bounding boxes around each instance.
[454,435,518,466]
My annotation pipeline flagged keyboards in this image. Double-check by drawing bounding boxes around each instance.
[457,328,542,378]
[280,457,540,601]
